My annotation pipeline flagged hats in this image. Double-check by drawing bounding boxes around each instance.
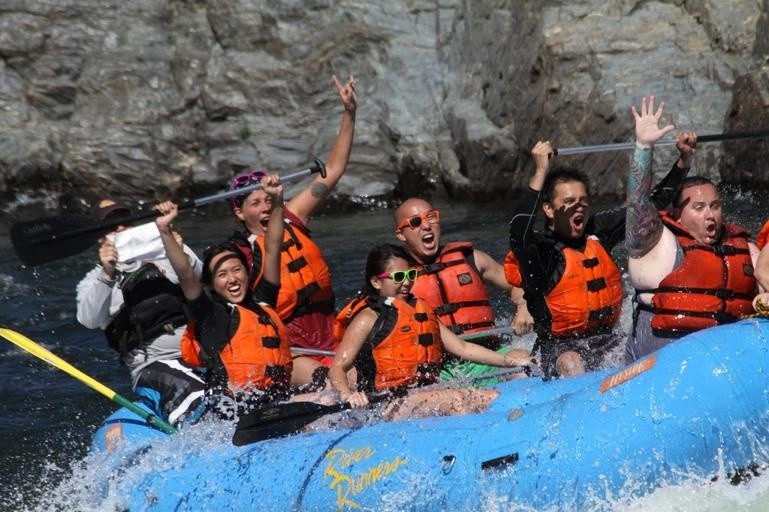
[96,199,131,220]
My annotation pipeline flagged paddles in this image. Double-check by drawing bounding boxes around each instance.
[0,328,179,435]
[233,358,537,446]
[10,158,326,266]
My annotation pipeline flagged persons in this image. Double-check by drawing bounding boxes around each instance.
[624,93,768,362]
[152,173,295,418]
[507,132,697,376]
[226,76,361,391]
[74,199,207,431]
[324,197,533,422]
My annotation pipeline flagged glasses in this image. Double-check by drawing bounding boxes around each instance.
[397,210,440,229]
[377,269,418,283]
[231,172,267,208]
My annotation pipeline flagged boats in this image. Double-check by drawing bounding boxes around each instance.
[88,313,767,511]
